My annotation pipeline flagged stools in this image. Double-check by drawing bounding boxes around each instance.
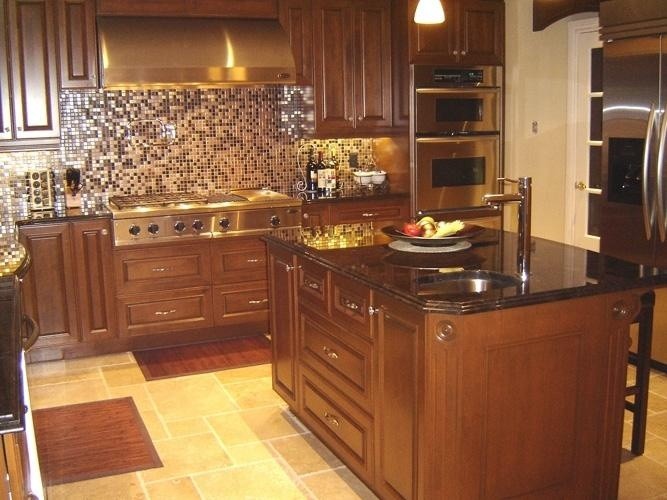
[621,292,655,455]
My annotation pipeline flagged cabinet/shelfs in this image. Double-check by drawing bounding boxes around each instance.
[114,233,269,344]
[313,0,393,133]
[275,0,312,89]
[0,273,45,499]
[266,241,429,500]
[16,216,117,352]
[1,0,60,151]
[394,0,503,69]
[61,0,99,91]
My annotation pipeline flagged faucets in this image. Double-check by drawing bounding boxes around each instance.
[128,117,164,146]
[482,177,532,275]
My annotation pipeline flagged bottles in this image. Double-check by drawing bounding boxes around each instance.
[307,150,318,192]
[324,149,341,193]
[315,150,326,191]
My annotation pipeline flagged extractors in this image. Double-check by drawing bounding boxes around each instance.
[94,1,300,93]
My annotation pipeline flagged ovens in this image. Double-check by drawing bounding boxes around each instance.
[413,63,503,218]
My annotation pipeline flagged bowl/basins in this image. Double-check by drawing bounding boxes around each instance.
[350,170,387,186]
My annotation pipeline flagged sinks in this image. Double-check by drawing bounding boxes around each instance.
[414,268,520,295]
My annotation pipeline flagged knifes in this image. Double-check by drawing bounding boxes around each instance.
[67,167,79,186]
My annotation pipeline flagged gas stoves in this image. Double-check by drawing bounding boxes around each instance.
[99,188,303,245]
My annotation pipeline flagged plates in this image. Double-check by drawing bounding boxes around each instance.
[380,249,487,272]
[380,222,487,246]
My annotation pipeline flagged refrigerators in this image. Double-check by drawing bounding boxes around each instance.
[598,0,667,271]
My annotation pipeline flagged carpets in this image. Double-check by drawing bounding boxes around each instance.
[32,394,164,487]
[130,336,271,382]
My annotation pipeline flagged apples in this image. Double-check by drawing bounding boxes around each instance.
[421,223,435,237]
[403,224,420,236]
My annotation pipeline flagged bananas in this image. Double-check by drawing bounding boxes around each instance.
[416,216,438,228]
